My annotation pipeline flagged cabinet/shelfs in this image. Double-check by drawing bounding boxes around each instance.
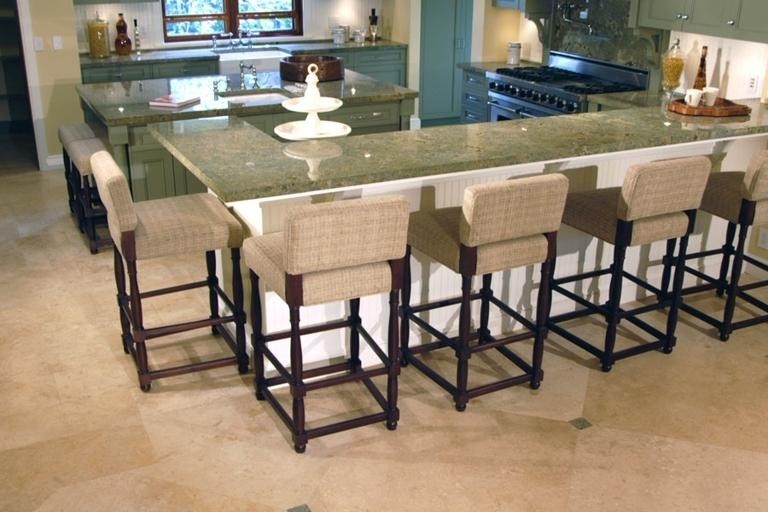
[627,0,768,44]
[313,49,406,87]
[126,99,400,203]
[82,60,218,84]
[494,0,552,17]
[460,72,489,124]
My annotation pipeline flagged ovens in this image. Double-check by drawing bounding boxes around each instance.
[486,90,589,123]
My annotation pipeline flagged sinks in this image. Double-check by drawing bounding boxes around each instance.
[216,49,292,74]
[219,92,293,107]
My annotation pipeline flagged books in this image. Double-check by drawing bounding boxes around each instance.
[149,94,200,107]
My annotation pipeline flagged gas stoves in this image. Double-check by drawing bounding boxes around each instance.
[484,63,647,114]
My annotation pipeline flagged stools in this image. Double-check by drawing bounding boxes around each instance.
[58,122,107,218]
[67,136,113,254]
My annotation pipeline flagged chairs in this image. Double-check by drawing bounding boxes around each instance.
[544,154,712,372]
[659,149,768,341]
[401,172,570,412]
[89,150,249,391]
[242,195,412,453]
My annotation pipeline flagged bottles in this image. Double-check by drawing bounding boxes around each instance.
[85,9,143,59]
[659,36,685,108]
[692,45,711,100]
[328,7,382,48]
[505,40,521,65]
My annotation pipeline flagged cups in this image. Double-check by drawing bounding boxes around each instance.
[682,87,703,108]
[700,87,720,108]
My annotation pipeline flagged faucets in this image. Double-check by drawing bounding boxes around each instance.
[237,27,245,42]
[238,62,257,87]
